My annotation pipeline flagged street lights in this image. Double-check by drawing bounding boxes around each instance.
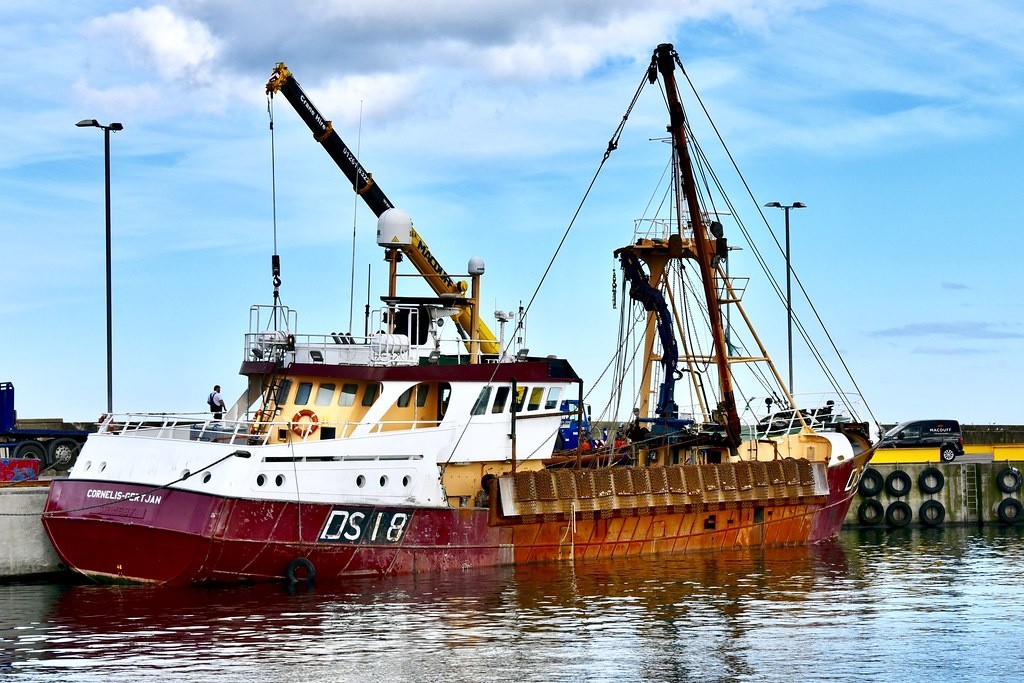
[764,201,807,408]
[75,120,124,412]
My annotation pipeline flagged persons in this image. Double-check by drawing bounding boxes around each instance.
[207,385,227,420]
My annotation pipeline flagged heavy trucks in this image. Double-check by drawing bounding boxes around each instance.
[0,382,98,471]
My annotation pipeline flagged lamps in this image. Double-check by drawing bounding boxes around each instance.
[428,350,441,365]
[251,348,265,361]
[310,350,324,364]
[515,349,529,363]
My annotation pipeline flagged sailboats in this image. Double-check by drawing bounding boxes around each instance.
[40,42,886,585]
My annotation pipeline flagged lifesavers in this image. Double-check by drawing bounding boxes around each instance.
[998,497,1024,522]
[919,499,945,525]
[857,468,884,496]
[884,470,912,496]
[918,467,944,494]
[288,556,315,581]
[886,501,912,526]
[251,409,263,433]
[858,498,884,524]
[996,466,1023,493]
[292,409,318,436]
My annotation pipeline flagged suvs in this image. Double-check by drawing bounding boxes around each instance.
[878,420,965,462]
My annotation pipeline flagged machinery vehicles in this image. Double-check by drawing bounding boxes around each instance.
[265,61,592,449]
